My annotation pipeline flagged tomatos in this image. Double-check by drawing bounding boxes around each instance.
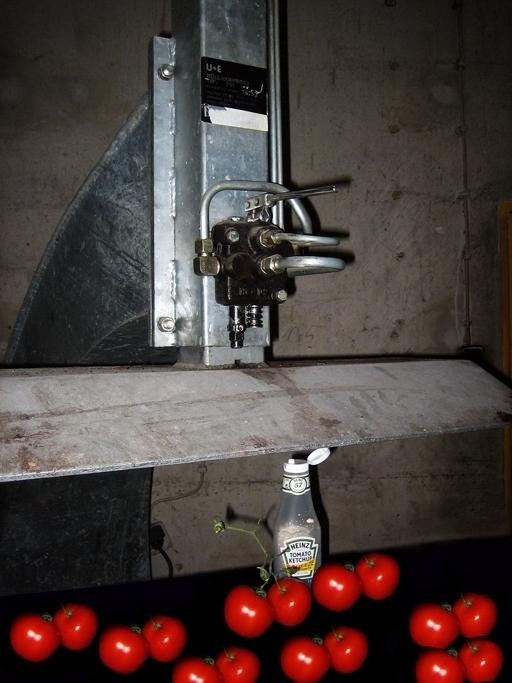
[267,576,311,626]
[214,646,260,682]
[460,640,502,682]
[141,614,186,663]
[224,585,274,639]
[11,604,62,662]
[324,626,368,674]
[99,615,150,673]
[53,604,97,650]
[452,593,497,638]
[415,650,463,682]
[280,636,329,682]
[408,604,459,649]
[311,562,363,611]
[173,656,221,683]
[356,555,399,600]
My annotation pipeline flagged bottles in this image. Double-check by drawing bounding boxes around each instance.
[271,448,332,587]
